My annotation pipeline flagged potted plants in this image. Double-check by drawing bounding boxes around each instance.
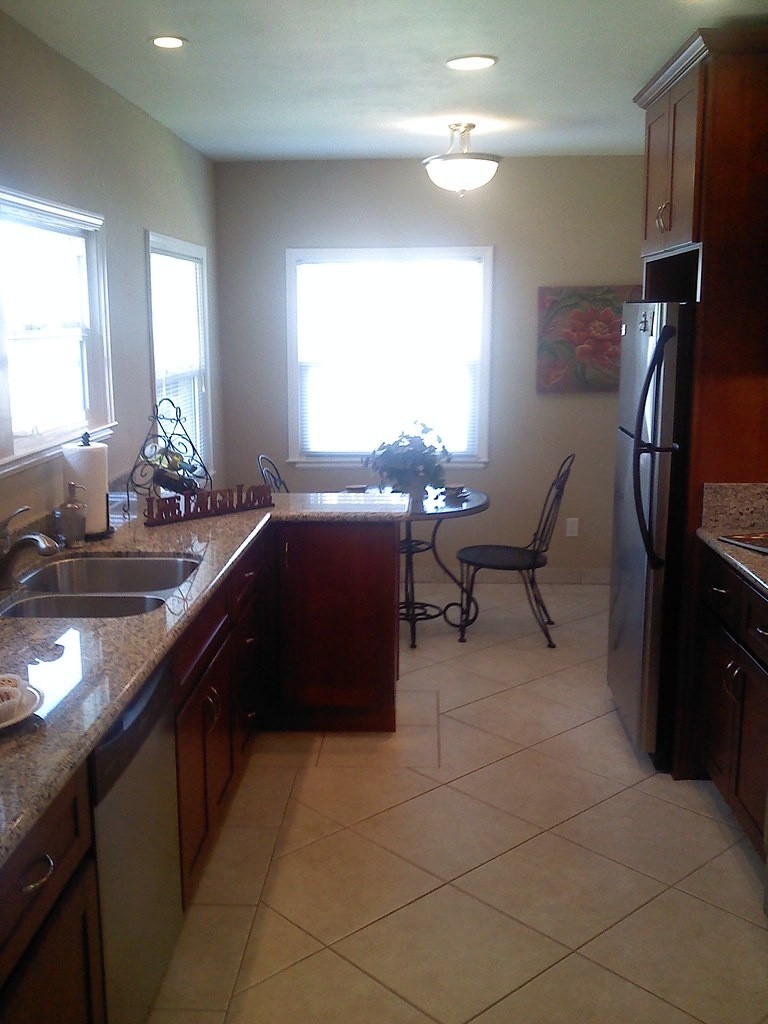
[364,423,452,498]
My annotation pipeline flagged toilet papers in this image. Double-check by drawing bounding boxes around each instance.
[59,442,108,535]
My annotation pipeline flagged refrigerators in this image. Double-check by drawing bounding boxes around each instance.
[611,304,690,774]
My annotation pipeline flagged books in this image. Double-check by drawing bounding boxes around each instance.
[718,531,768,554]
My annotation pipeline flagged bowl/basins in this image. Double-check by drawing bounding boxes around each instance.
[345,485,368,493]
[444,484,465,497]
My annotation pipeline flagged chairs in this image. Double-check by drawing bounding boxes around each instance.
[457,453,576,649]
[258,454,289,493]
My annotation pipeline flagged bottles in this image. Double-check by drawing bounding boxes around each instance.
[144,443,195,474]
[153,470,210,497]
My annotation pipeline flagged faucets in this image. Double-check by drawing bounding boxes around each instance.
[0,505,59,591]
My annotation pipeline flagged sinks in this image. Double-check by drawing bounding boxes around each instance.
[0,593,177,620]
[12,550,210,594]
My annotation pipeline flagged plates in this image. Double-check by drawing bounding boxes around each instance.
[0,684,44,728]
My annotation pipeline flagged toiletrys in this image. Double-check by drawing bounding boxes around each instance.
[55,482,87,549]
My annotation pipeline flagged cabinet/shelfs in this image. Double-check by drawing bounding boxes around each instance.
[275,522,397,732]
[1,758,106,1024]
[172,577,233,912]
[229,538,264,774]
[699,549,768,867]
[640,65,704,258]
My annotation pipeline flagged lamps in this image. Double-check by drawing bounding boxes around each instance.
[421,123,501,197]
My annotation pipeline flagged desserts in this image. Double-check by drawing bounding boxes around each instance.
[0,678,19,704]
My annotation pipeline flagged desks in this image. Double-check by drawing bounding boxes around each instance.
[340,486,490,648]
